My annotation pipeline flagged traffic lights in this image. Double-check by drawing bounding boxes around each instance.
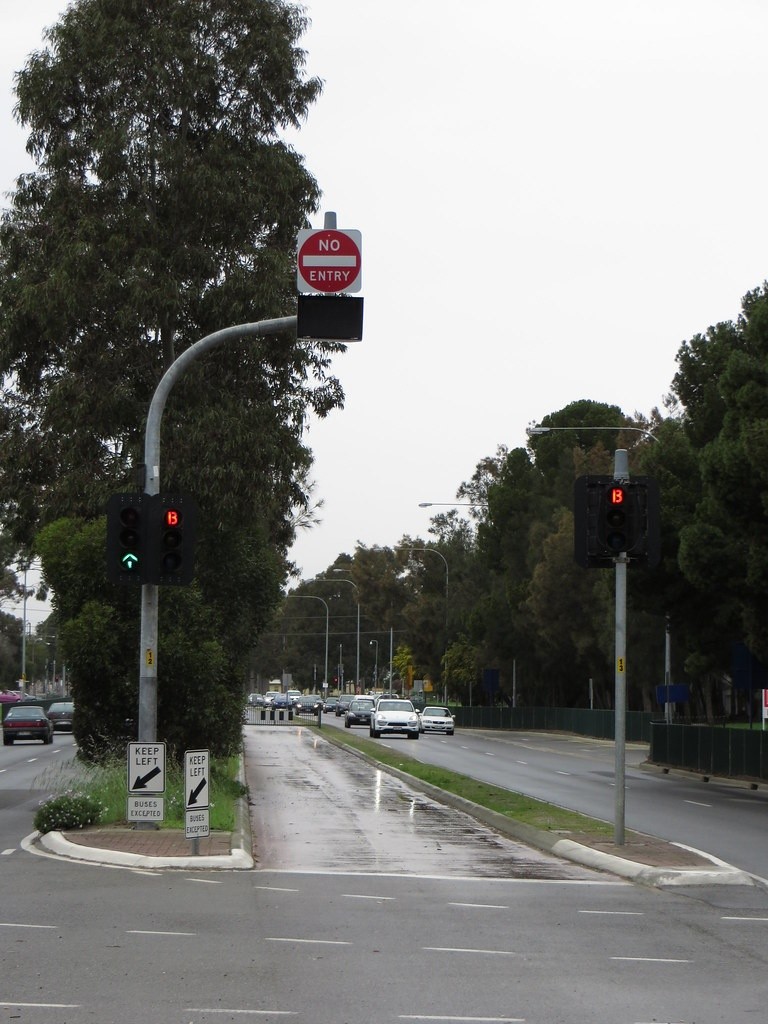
[151,493,197,587]
[108,491,154,586]
[333,677,338,683]
[297,295,363,342]
[598,482,644,554]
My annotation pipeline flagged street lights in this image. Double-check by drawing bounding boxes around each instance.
[526,426,677,722]
[374,548,449,706]
[418,502,518,706]
[332,569,395,699]
[283,594,329,699]
[307,579,360,686]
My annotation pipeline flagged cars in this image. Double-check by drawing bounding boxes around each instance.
[354,694,399,699]
[0,690,21,703]
[305,695,324,711]
[323,697,340,714]
[418,706,456,735]
[271,694,293,711]
[294,696,320,716]
[343,699,376,729]
[47,703,76,733]
[13,691,36,702]
[248,693,263,706]
[2,705,54,745]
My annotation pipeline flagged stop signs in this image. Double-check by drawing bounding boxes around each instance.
[297,228,363,294]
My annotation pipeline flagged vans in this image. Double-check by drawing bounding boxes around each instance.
[286,690,302,711]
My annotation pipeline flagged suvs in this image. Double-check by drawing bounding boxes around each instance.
[369,699,421,740]
[336,695,355,718]
[262,692,281,708]
